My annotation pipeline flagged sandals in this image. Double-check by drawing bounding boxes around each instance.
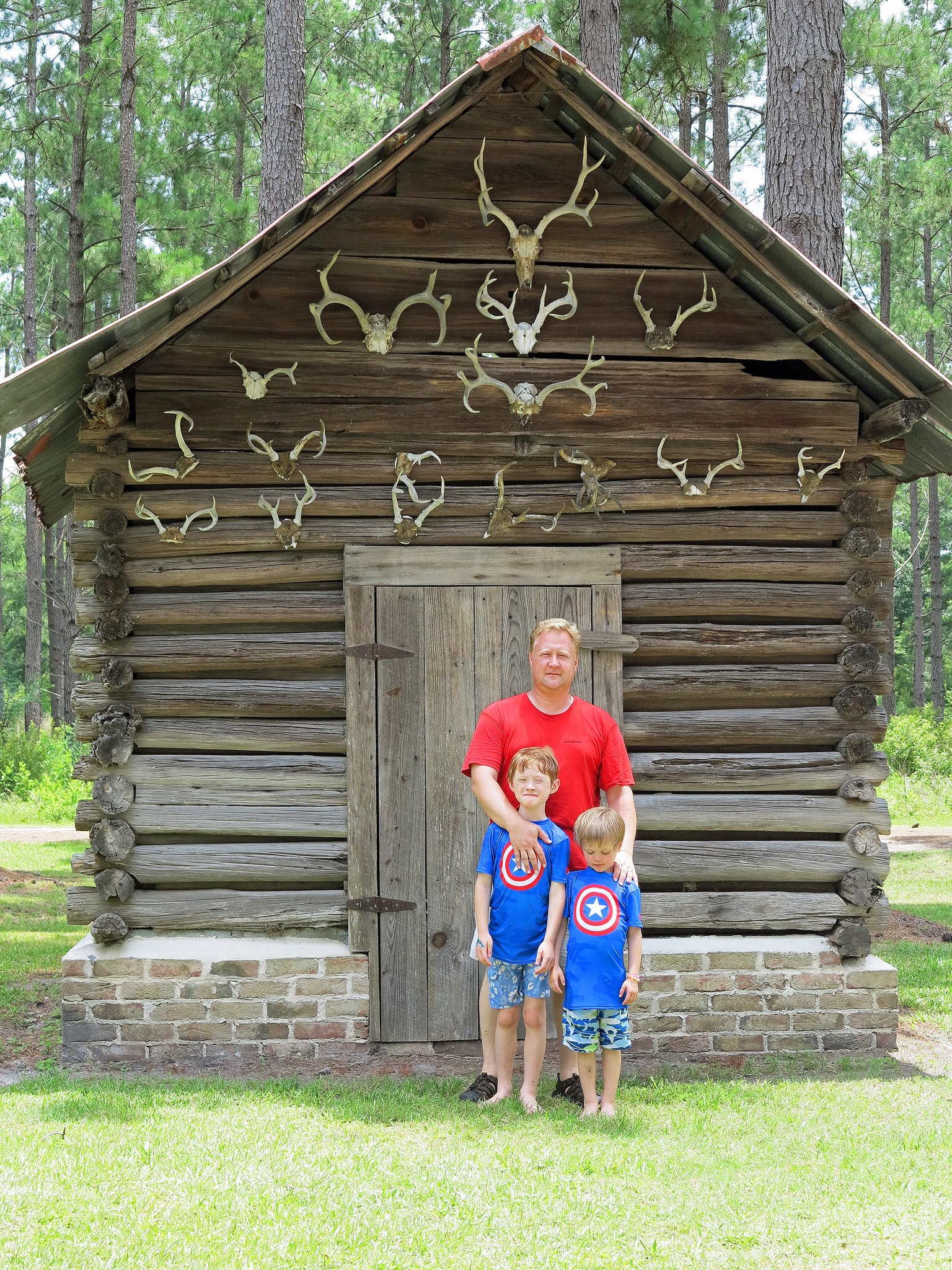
[459,1072,497,1103]
[552,1072,603,1111]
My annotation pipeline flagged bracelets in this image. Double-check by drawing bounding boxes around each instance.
[626,972,641,982]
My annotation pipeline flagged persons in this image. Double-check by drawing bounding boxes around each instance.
[462,618,639,1110]
[548,806,643,1119]
[469,745,570,1114]
